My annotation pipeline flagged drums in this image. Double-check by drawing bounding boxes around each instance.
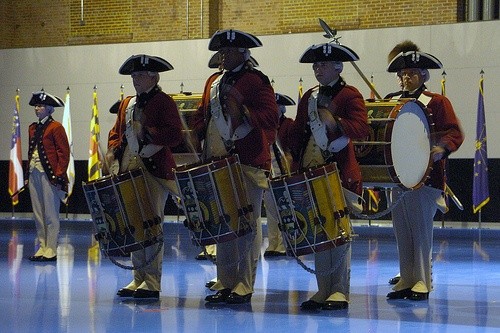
[83,166,164,258]
[267,162,353,258]
[352,99,434,191]
[172,153,257,247]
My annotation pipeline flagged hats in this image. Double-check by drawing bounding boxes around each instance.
[386,50,444,73]
[207,28,263,51]
[28,89,66,108]
[274,92,296,106]
[207,50,259,68]
[118,53,174,75]
[299,42,361,62]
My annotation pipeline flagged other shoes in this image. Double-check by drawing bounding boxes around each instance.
[30,254,57,262]
[195,253,213,259]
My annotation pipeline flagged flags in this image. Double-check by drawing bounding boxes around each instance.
[88,91,102,181]
[440,80,449,213]
[62,92,75,205]
[8,95,23,205]
[368,82,380,212]
[298,84,303,104]
[472,78,490,213]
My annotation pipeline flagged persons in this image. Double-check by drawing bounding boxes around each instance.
[24,90,71,262]
[102,28,464,310]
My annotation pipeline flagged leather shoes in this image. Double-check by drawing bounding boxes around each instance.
[322,301,348,310]
[389,276,401,284]
[224,292,253,304]
[409,292,430,300]
[132,287,160,301]
[386,287,412,299]
[205,281,217,288]
[204,289,230,304]
[300,300,325,310]
[116,288,134,299]
